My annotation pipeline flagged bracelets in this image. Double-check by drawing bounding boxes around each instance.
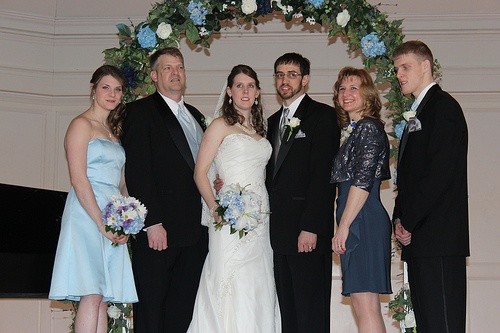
[210,204,219,217]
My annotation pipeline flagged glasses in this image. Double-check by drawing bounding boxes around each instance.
[273,72,303,79]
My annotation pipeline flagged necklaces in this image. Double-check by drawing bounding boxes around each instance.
[235,121,256,135]
[100,122,114,138]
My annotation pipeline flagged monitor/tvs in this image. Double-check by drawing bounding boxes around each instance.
[0,183,69,299]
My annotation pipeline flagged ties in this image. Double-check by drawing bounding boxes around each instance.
[275,108,291,163]
[177,105,200,164]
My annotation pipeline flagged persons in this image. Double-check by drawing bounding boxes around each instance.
[332,67,393,333]
[48,64,139,333]
[392,40,471,333]
[185,64,282,333]
[121,47,209,333]
[213,53,341,333]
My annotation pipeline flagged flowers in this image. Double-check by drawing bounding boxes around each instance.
[284,117,301,142]
[213,182,272,240]
[384,281,417,333]
[201,116,213,129]
[106,304,133,333]
[239,0,258,16]
[184,0,222,49]
[340,120,357,147]
[114,16,179,50]
[401,110,422,134]
[360,31,387,58]
[336,9,351,28]
[306,0,325,9]
[101,194,147,247]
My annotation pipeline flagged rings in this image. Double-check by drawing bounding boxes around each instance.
[338,245,342,248]
[309,247,312,250]
